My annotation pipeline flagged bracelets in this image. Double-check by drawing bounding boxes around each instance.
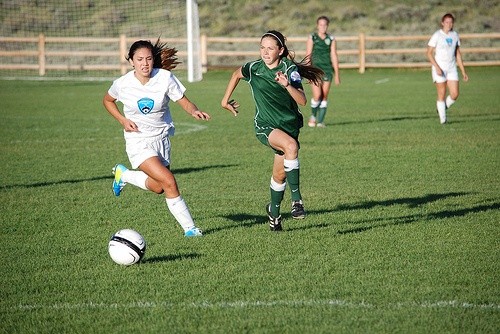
[285,83,290,87]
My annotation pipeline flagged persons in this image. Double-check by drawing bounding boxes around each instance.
[223,30,325,232]
[102,36,211,239]
[427,14,468,125]
[306,16,340,128]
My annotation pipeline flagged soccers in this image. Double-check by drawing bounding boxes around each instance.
[107,228,146,265]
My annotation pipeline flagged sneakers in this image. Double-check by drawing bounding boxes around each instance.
[265,203,282,232]
[290,200,306,218]
[308,118,316,127]
[183,227,203,238]
[112,164,128,195]
